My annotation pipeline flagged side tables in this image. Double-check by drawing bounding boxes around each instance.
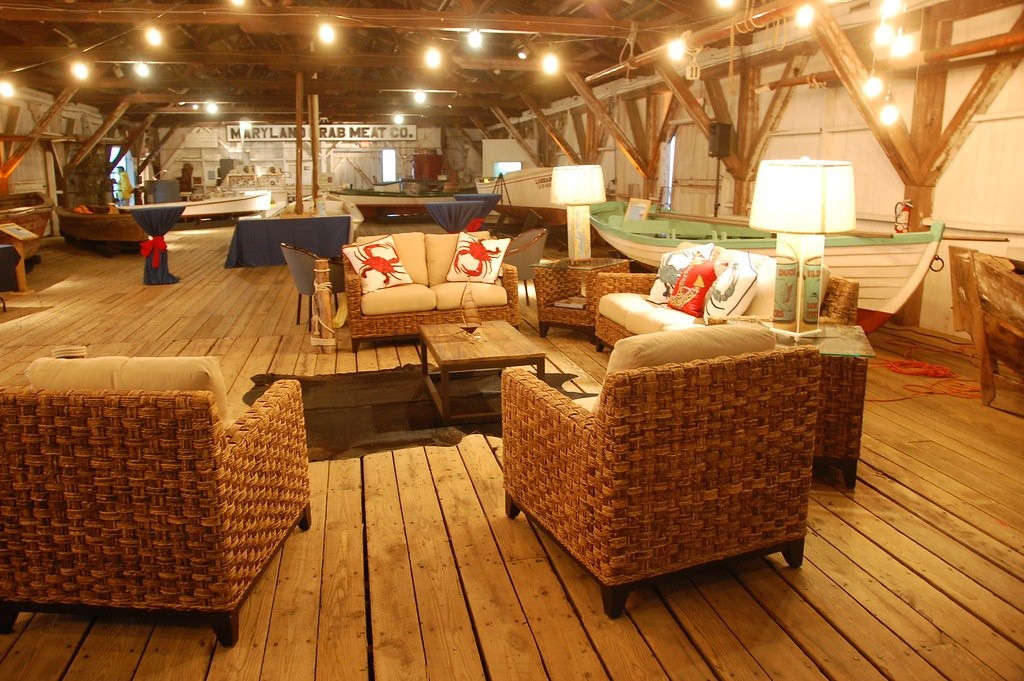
[796,324,875,489]
[530,258,635,345]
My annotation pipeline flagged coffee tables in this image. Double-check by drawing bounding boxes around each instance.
[420,321,545,429]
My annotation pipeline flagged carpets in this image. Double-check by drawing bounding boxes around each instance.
[242,364,601,462]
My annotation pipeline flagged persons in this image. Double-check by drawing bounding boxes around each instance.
[116,165,134,206]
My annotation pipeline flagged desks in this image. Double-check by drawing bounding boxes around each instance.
[226,214,351,269]
[0,245,22,312]
[424,200,485,235]
[129,201,190,286]
[454,193,502,234]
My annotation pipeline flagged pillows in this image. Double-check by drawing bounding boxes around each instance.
[647,243,715,304]
[342,233,413,295]
[445,231,512,284]
[667,253,728,318]
[702,249,758,325]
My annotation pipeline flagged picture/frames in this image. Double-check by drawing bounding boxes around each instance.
[624,198,651,222]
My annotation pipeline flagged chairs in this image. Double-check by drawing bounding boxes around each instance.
[0,356,314,648]
[279,243,345,334]
[502,319,824,620]
[504,228,549,304]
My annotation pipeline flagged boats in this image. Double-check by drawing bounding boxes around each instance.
[0,191,56,259]
[475,166,606,226]
[590,201,944,334]
[56,206,147,247]
[265,188,364,236]
[322,189,472,217]
[115,190,271,226]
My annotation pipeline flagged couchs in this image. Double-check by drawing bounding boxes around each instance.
[594,242,860,353]
[341,231,520,354]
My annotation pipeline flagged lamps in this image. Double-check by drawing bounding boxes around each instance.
[517,47,531,59]
[550,166,608,263]
[113,64,125,77]
[749,157,856,344]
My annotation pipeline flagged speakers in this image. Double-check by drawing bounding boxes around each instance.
[708,121,731,158]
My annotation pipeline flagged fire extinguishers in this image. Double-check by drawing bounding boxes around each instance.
[894,198,913,233]
